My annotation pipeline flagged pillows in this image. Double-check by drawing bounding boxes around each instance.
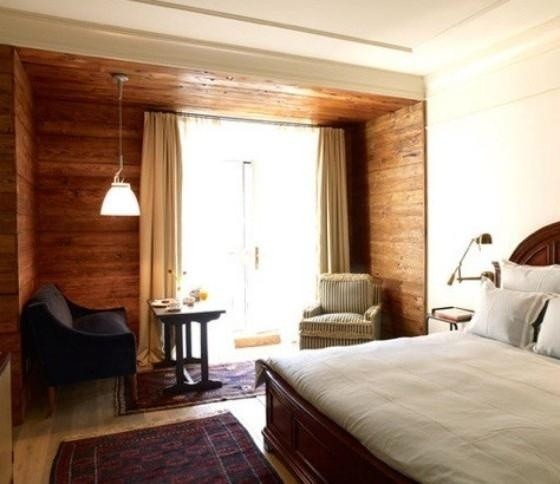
[499,258,560,294]
[524,292,560,360]
[465,276,553,350]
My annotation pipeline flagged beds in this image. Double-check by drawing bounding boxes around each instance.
[255,220,560,483]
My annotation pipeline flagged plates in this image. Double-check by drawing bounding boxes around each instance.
[151,298,175,307]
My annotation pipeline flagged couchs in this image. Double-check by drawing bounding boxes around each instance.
[20,281,138,417]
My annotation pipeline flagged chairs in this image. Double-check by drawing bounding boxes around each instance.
[299,271,383,349]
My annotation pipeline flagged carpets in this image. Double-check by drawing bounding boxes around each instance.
[115,359,266,418]
[48,411,285,484]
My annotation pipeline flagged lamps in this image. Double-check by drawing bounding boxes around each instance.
[100,71,141,218]
[447,233,493,286]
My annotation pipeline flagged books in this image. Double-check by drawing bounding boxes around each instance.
[435,307,472,322]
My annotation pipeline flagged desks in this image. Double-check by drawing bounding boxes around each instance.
[147,295,226,396]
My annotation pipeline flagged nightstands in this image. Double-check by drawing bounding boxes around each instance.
[424,307,475,336]
